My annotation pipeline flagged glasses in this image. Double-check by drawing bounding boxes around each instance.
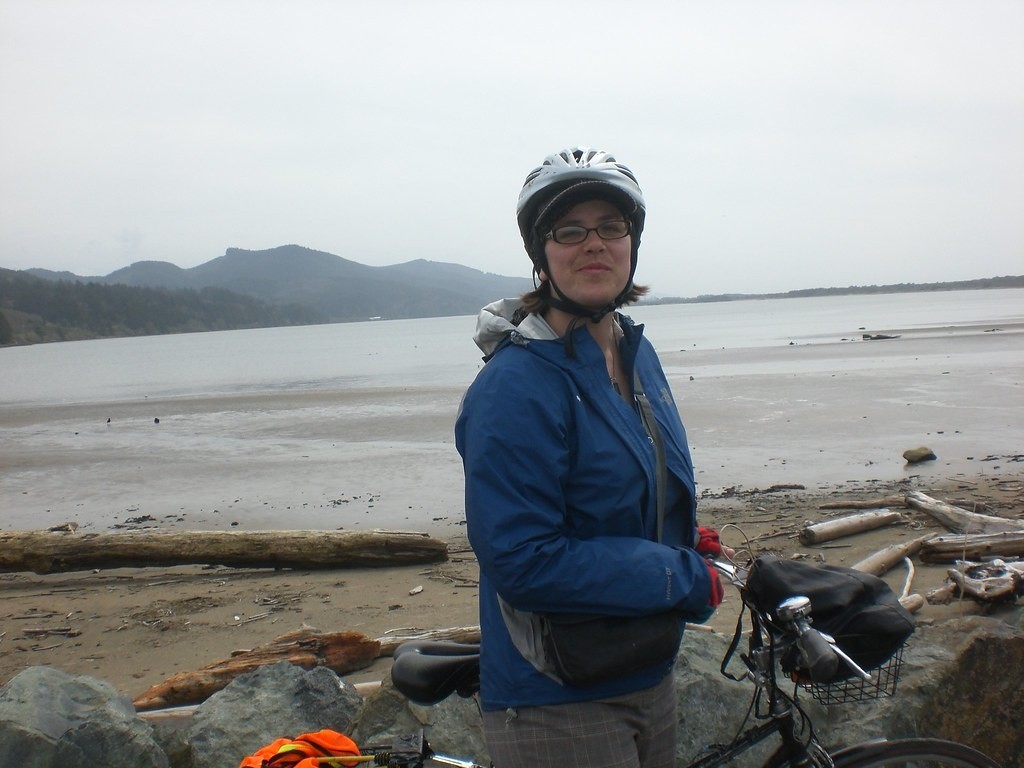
[544,220,632,245]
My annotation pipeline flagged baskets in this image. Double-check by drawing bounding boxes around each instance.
[781,640,909,706]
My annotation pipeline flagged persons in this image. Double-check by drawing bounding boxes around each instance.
[454,151,734,768]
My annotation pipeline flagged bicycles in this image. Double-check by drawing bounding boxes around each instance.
[350,553,1003,768]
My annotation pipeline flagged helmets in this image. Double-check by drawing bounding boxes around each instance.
[517,150,645,247]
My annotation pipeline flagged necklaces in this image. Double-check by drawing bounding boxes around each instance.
[604,321,622,398]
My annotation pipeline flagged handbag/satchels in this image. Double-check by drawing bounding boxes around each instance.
[720,554,916,718]
